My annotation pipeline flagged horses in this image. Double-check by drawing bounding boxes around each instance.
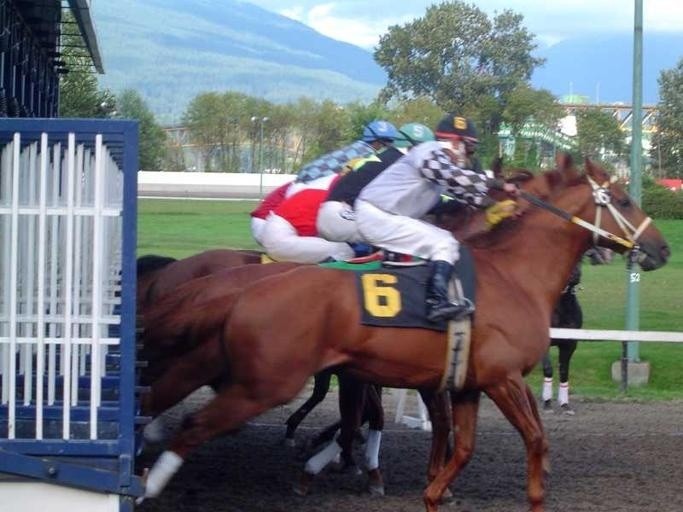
[114,147,671,512]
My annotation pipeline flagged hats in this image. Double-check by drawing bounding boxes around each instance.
[361,120,399,144]
[393,122,436,148]
[435,113,481,145]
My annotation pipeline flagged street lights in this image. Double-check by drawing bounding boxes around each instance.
[251,116,269,193]
[101,102,119,119]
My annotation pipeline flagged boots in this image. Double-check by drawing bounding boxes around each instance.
[422,259,466,323]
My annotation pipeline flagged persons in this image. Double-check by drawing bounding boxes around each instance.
[249,112,522,322]
